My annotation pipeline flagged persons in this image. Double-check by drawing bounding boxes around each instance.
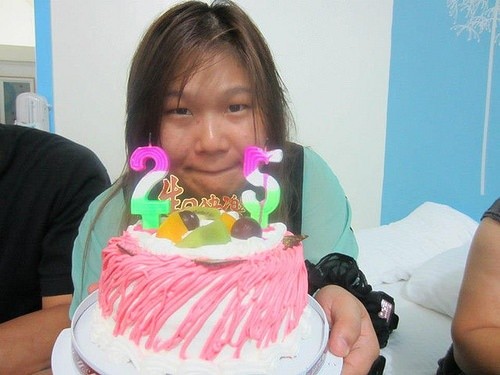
[68,0,380,375]
[1,124,112,375]
[452,198,499,373]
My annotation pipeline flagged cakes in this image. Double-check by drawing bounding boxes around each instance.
[88,131,311,373]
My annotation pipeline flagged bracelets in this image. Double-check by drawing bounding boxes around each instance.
[305,252,399,347]
